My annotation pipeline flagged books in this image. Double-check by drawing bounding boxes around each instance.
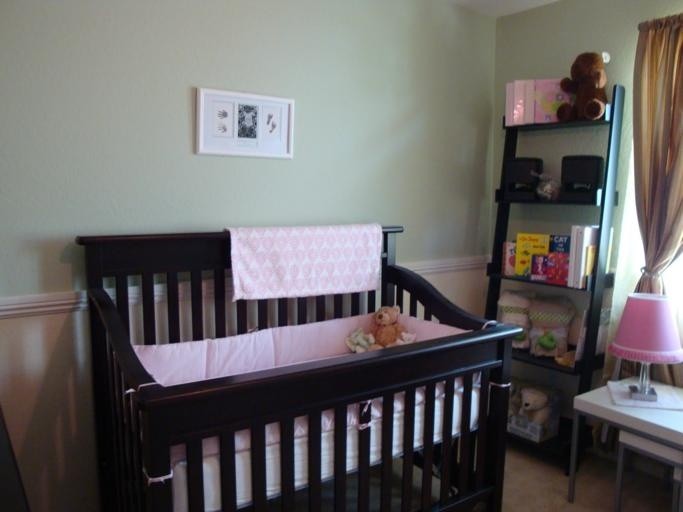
[502,221,600,289]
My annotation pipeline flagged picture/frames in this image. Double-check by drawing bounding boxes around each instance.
[195,87,296,159]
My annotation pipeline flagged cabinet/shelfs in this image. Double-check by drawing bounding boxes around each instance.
[480,84,627,474]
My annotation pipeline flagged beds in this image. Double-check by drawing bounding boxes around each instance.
[73,225,525,512]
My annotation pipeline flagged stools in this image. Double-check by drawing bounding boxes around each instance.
[613,431,683,511]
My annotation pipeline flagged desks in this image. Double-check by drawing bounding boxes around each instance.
[567,375,683,505]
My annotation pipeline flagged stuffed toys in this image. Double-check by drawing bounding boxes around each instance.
[530,168,562,201]
[557,50,609,122]
[393,331,418,346]
[517,386,553,423]
[369,303,408,351]
[345,328,376,354]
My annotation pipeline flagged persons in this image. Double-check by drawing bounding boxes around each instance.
[239,103,257,137]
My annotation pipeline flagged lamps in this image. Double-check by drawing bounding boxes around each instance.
[608,292,683,401]
